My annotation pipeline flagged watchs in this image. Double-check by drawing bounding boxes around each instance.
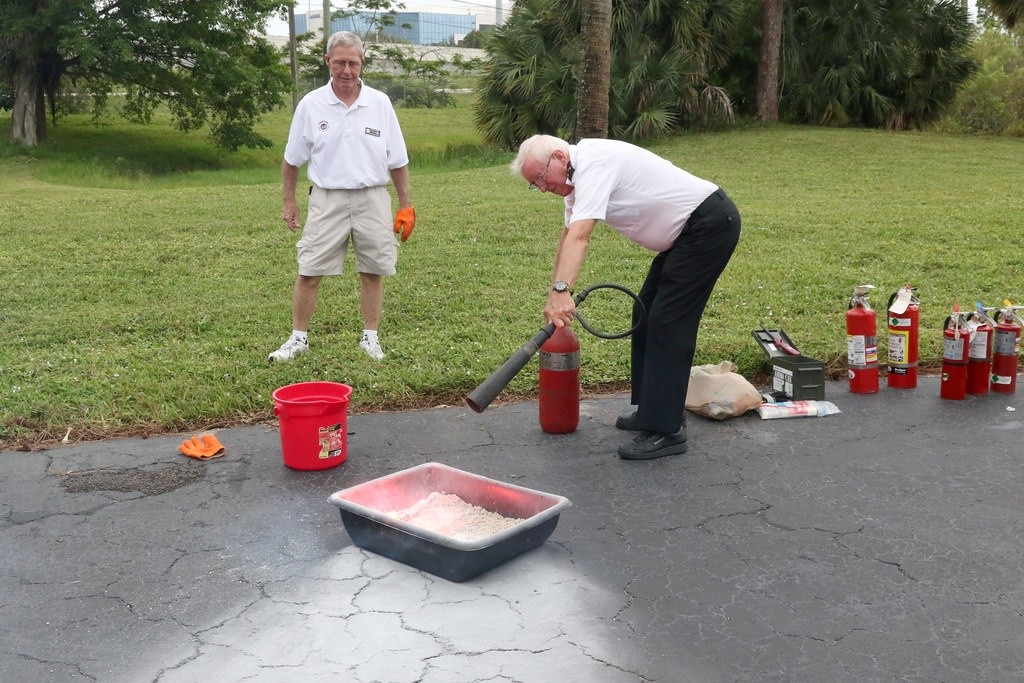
[552,280,573,296]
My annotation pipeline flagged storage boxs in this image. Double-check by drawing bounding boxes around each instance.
[327,461,573,583]
[751,328,827,401]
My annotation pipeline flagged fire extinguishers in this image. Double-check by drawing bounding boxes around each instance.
[969,299,1024,396]
[539,316,580,434]
[845,284,880,394]
[886,282,921,389]
[939,304,972,400]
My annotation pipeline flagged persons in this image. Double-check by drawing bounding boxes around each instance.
[510,133,741,460]
[268,30,415,361]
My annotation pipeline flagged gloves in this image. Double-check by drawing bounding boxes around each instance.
[394,208,415,242]
[177,433,226,461]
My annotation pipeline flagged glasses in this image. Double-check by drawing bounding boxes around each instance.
[529,156,552,190]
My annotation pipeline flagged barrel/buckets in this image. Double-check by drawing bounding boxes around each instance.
[272,381,353,470]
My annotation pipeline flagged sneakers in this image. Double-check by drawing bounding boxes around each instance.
[615,411,686,429]
[618,426,688,459]
[359,334,386,363]
[268,335,309,365]
